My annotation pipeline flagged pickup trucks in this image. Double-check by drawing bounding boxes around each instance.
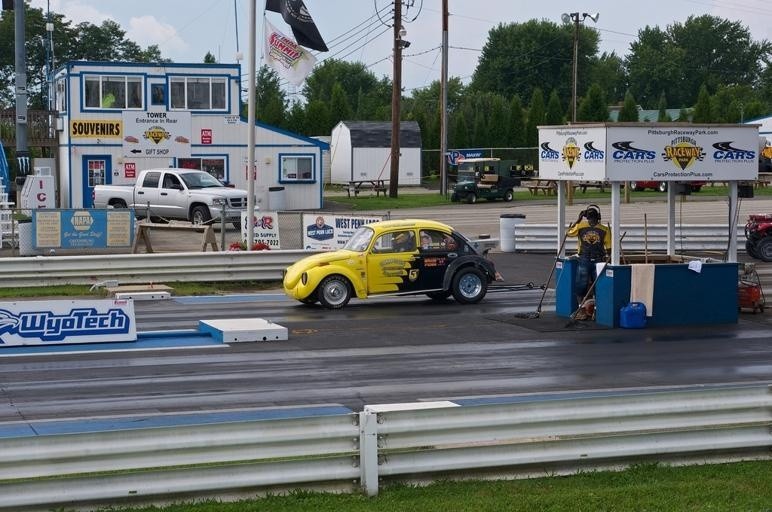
[92,167,260,232]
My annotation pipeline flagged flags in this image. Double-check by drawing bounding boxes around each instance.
[264,1,330,54]
[260,13,317,92]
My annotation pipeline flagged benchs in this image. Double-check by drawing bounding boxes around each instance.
[342,186,388,197]
[579,184,606,193]
[526,186,554,196]
[572,185,579,193]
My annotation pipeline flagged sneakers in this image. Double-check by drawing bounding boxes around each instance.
[571,307,587,319]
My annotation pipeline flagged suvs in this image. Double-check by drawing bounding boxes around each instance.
[745,213,772,262]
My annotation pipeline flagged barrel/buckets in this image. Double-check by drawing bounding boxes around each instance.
[620,301,647,328]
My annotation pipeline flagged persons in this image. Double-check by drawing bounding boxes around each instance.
[566,208,613,320]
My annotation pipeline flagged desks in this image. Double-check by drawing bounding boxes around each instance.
[530,177,559,196]
[348,180,386,197]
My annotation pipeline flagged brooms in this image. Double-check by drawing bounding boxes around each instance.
[564,231,626,331]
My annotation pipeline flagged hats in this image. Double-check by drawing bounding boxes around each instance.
[587,208,598,220]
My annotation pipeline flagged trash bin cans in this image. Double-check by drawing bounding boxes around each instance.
[555,256,592,318]
[268,187,286,212]
[594,262,632,329]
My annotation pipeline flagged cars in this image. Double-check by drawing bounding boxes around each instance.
[631,181,708,192]
[283,218,504,310]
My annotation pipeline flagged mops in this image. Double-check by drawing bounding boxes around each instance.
[515,222,572,319]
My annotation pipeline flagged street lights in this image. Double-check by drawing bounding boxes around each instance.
[562,12,599,121]
